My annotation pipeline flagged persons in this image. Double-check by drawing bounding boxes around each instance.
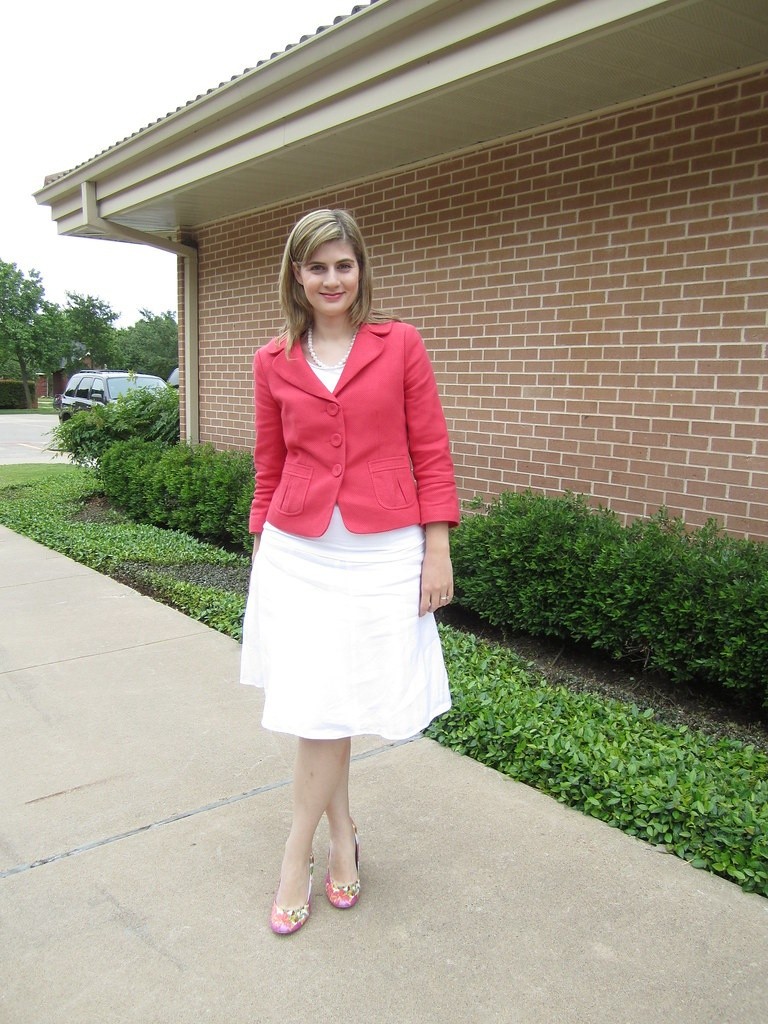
[240,209,460,934]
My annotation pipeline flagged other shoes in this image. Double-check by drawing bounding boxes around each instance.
[325,818,360,909]
[269,852,314,934]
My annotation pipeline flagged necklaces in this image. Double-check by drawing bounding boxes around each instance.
[308,323,358,368]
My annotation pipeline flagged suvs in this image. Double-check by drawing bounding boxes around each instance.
[59,369,180,428]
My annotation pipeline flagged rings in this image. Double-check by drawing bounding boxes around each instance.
[440,597,447,599]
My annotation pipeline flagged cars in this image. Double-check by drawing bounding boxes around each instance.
[53,393,63,410]
[166,368,179,393]
